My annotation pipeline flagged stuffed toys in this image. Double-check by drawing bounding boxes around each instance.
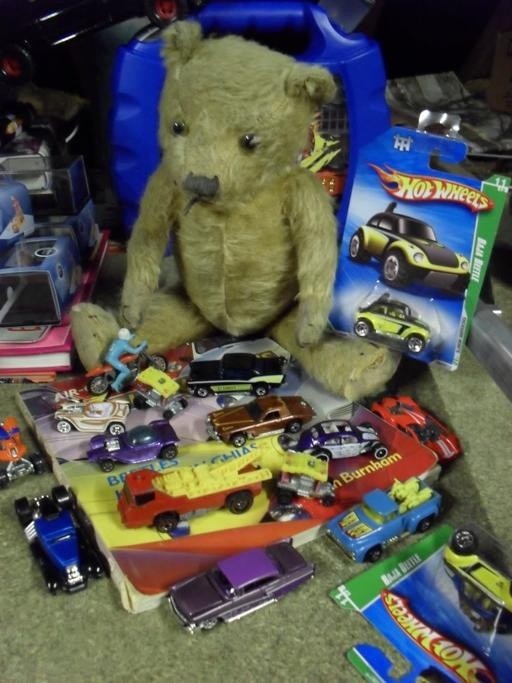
[65,15,403,405]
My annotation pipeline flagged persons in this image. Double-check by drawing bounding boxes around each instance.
[103,328,148,393]
